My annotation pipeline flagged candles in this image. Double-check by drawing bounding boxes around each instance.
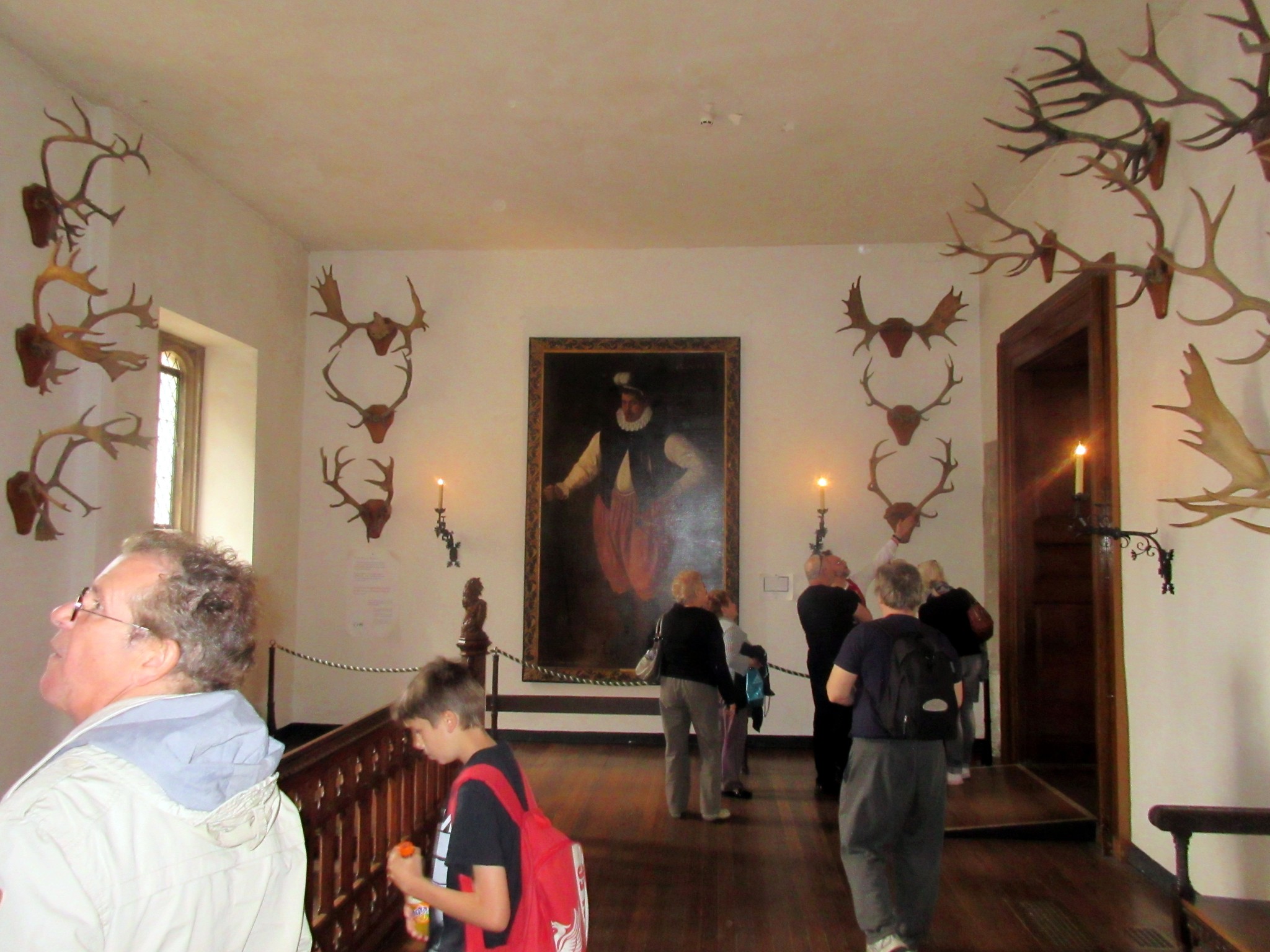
[438,479,444,508]
[818,477,826,508]
[1075,445,1086,493]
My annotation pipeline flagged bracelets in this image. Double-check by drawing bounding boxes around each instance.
[892,534,903,543]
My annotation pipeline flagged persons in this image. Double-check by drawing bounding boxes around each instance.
[916,561,993,786]
[703,590,771,801]
[385,657,591,952]
[635,570,735,821]
[821,514,917,608]
[0,528,313,952]
[826,560,965,952]
[797,555,872,801]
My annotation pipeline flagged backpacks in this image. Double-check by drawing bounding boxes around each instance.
[861,619,959,741]
[446,759,589,952]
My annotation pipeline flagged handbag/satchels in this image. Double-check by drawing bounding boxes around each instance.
[635,613,666,685]
[958,587,994,641]
[746,666,766,709]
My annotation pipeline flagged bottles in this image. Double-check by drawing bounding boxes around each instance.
[398,840,431,935]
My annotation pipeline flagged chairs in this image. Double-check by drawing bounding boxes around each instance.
[1148,803,1270,951]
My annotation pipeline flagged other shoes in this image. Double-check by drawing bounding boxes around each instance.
[946,771,964,786]
[721,787,752,799]
[814,783,840,801]
[962,768,971,779]
[702,808,731,821]
[670,811,685,818]
[865,932,918,952]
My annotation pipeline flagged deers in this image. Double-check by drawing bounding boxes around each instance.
[835,275,968,545]
[310,264,429,542]
[938,0,1270,539]
[6,96,159,542]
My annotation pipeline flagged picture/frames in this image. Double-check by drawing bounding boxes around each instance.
[519,335,738,685]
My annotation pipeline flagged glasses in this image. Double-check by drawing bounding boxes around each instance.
[70,587,181,650]
[811,553,823,573]
[835,558,844,566]
